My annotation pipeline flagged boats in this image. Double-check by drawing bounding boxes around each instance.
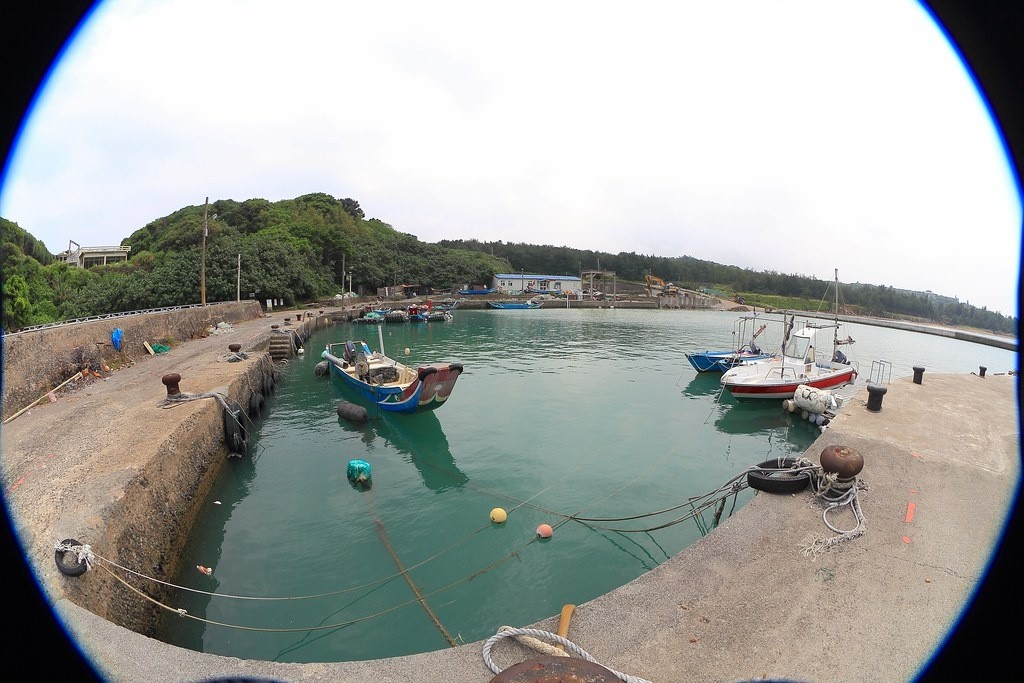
[320,325,464,416]
[720,267,860,401]
[457,285,501,295]
[351,299,460,326]
[683,302,801,374]
[487,299,545,310]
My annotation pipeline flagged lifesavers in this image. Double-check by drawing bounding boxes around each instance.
[249,392,266,420]
[746,457,817,495]
[295,328,311,346]
[225,403,249,456]
[355,361,368,376]
[262,376,275,399]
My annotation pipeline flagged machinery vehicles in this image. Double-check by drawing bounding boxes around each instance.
[643,273,682,298]
